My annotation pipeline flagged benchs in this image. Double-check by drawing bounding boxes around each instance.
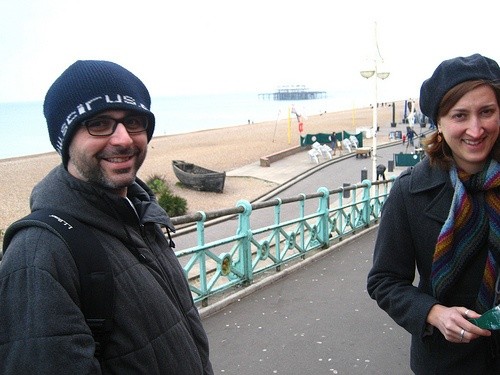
[260,145,302,167]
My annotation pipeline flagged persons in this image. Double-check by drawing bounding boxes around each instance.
[367,52,500,375]
[376,164,386,181]
[403,125,427,152]
[330,131,337,154]
[0,60,216,375]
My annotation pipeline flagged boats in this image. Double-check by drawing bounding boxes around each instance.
[172,160,226,193]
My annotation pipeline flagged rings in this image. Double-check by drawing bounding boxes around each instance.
[460,329,465,336]
[464,310,470,317]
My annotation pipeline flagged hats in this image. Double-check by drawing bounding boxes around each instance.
[43,60,156,169]
[418,53,499,127]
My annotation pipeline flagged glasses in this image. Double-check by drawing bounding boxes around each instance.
[81,111,151,137]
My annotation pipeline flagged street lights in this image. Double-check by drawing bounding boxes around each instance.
[360,45,391,196]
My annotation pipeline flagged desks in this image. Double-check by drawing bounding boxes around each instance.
[355,148,372,158]
